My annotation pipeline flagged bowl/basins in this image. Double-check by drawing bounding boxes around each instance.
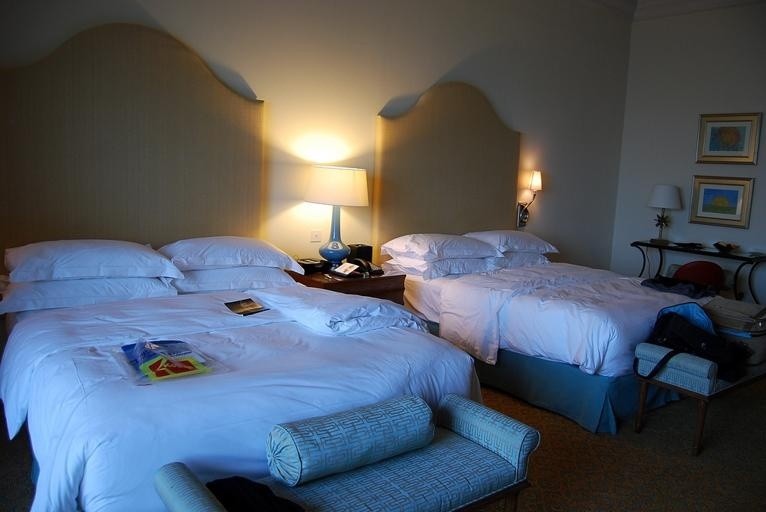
[712,244,738,252]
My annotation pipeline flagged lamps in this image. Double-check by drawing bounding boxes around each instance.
[646,184,685,247]
[515,168,546,228]
[297,162,370,268]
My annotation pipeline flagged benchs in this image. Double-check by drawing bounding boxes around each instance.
[151,393,544,512]
[632,317,765,454]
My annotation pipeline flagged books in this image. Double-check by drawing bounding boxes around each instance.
[221,296,270,318]
[121,339,213,384]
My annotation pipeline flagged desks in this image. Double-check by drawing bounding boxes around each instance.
[630,238,765,306]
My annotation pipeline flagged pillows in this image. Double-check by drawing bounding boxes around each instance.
[156,234,307,278]
[170,266,298,295]
[380,232,505,262]
[458,229,561,255]
[3,240,187,283]
[501,252,554,270]
[380,257,507,282]
[0,272,181,317]
[259,394,434,489]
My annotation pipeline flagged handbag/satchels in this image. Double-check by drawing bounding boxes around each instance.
[632,302,755,384]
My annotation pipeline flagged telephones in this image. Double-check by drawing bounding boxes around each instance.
[351,258,384,275]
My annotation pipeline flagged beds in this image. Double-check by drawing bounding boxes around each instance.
[1,21,481,512]
[372,81,730,440]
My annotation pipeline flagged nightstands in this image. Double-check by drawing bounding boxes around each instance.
[284,254,408,309]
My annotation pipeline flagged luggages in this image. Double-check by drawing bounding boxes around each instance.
[702,298,766,367]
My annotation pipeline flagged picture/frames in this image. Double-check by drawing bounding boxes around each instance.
[695,112,763,164]
[688,174,754,230]
